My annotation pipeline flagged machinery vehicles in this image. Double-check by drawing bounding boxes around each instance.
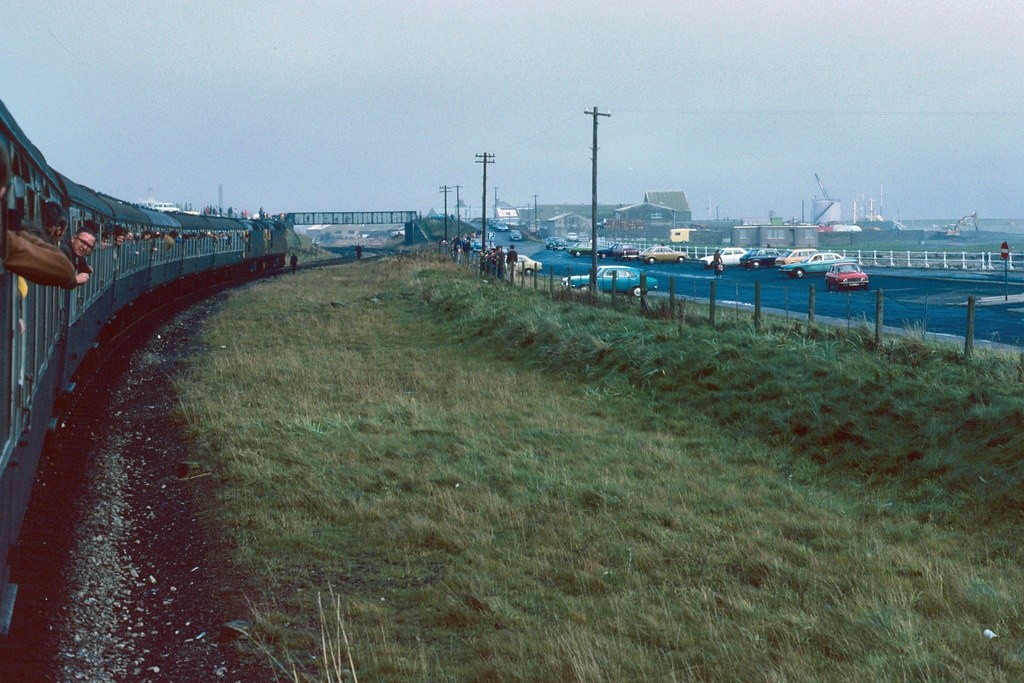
[945,212,978,236]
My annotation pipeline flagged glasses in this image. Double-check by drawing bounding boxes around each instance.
[76,237,93,248]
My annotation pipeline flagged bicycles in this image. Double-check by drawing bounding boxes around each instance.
[714,262,724,281]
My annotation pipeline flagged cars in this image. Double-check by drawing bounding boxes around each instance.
[561,265,658,298]
[470,236,509,255]
[638,246,688,264]
[824,263,869,291]
[774,249,819,268]
[510,230,522,241]
[615,243,641,261]
[699,248,748,268]
[740,248,780,269]
[564,241,593,257]
[546,232,587,252]
[596,244,619,260]
[503,255,543,276]
[488,218,509,232]
[779,253,858,278]
[154,202,178,214]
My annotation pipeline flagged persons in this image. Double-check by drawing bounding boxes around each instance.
[5,215,89,289]
[43,214,69,248]
[356,244,362,260]
[201,205,272,219]
[713,250,722,273]
[100,228,227,255]
[51,227,97,275]
[437,232,518,282]
[290,252,298,272]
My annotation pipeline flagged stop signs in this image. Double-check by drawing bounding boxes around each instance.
[1000,243,1009,259]
